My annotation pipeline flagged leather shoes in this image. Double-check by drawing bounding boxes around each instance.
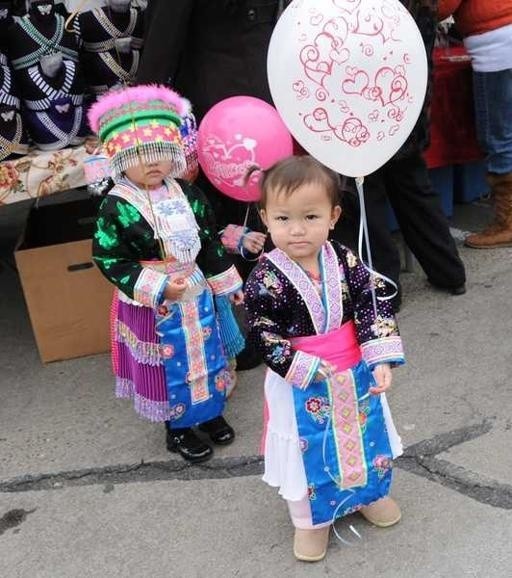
[166,427,213,463]
[199,419,237,446]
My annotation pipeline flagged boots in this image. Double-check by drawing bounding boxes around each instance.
[464,172,510,248]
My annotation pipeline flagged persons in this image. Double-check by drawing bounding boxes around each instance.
[178,130,268,375]
[432,1,511,251]
[243,155,406,563]
[365,4,468,313]
[81,83,238,463]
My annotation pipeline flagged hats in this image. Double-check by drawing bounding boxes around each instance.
[86,86,187,179]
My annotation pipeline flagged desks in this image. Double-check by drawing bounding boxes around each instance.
[342,44,495,271]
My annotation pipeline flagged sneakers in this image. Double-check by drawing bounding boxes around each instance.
[293,524,329,560]
[359,495,401,527]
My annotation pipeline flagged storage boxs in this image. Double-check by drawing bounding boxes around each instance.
[14,195,116,363]
[388,157,493,232]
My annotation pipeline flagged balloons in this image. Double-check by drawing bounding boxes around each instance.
[266,0,429,187]
[196,96,292,207]
